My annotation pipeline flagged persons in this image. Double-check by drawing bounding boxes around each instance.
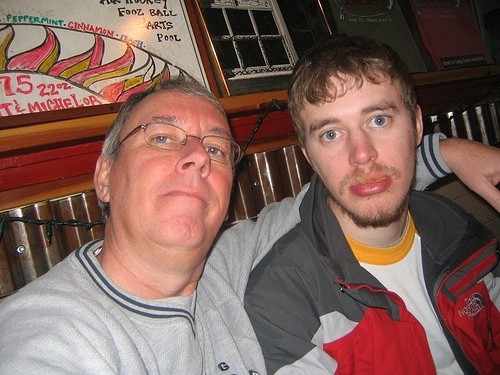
[243,35,500,374]
[1,77,500,375]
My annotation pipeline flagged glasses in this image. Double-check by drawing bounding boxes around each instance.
[111,120,244,169]
